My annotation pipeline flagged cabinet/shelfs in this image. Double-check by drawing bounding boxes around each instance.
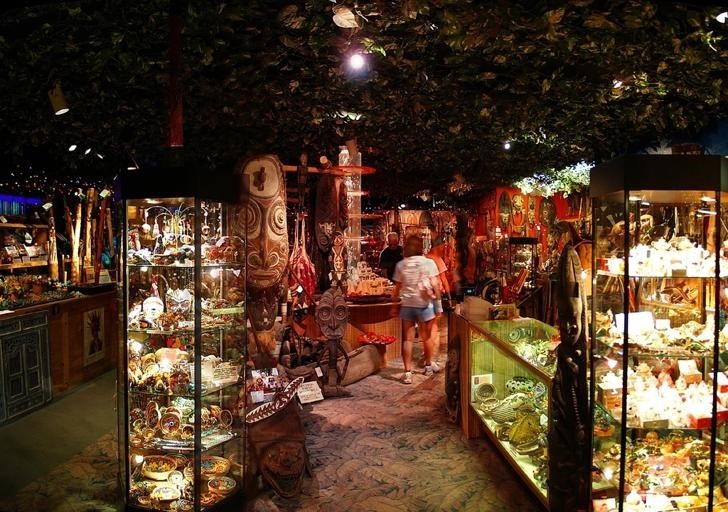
[0,221,49,270]
[114,162,252,512]
[461,308,562,510]
[577,150,728,511]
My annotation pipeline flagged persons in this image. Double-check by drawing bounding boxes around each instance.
[379,232,403,282]
[390,235,442,384]
[417,253,452,369]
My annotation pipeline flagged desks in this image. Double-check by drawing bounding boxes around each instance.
[0,286,119,429]
[346,298,405,362]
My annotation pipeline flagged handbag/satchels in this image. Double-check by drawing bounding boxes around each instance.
[417,273,436,302]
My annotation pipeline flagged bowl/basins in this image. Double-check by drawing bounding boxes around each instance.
[136,453,236,509]
[479,398,501,416]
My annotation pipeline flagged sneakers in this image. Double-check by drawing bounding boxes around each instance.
[423,363,436,378]
[401,369,413,384]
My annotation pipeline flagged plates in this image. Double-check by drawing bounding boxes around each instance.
[475,382,497,401]
[131,400,193,448]
